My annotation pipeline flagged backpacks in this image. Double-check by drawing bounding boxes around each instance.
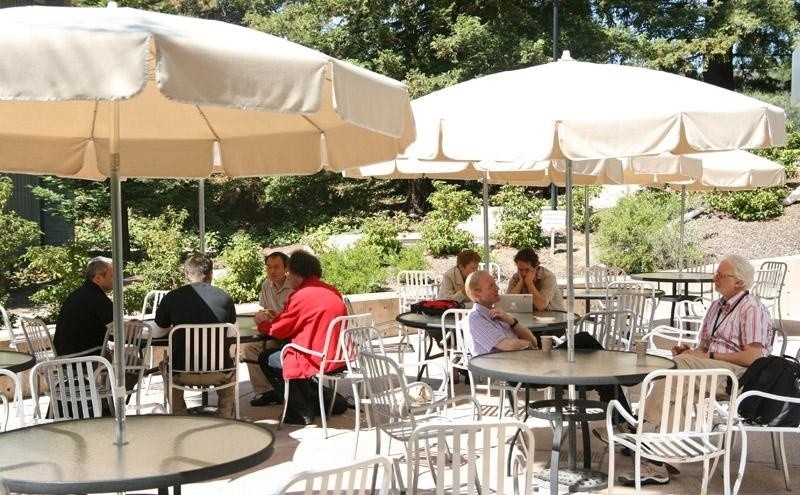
[410,299,463,317]
[732,354,796,423]
[315,380,348,416]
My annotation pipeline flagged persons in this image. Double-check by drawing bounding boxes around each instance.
[241,250,298,407]
[462,269,651,457]
[153,252,239,420]
[51,255,144,418]
[426,248,482,385]
[504,244,566,347]
[253,247,356,426]
[591,254,775,487]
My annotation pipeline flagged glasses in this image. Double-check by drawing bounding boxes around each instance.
[264,265,286,270]
[715,270,736,279]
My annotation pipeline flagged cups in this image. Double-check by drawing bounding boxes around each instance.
[259,309,270,317]
[635,341,648,357]
[541,336,552,352]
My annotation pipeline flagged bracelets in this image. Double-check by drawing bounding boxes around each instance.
[510,317,520,328]
[709,350,715,360]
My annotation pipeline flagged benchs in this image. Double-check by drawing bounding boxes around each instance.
[539,210,567,256]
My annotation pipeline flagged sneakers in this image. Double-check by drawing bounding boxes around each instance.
[616,461,672,485]
[592,422,637,447]
[250,393,280,407]
[279,414,314,425]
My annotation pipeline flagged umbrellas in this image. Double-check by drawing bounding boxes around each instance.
[342,157,703,276]
[643,149,789,328]
[394,47,787,471]
[582,153,705,318]
[0,0,418,494]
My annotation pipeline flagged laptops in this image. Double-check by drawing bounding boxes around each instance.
[494,293,533,314]
